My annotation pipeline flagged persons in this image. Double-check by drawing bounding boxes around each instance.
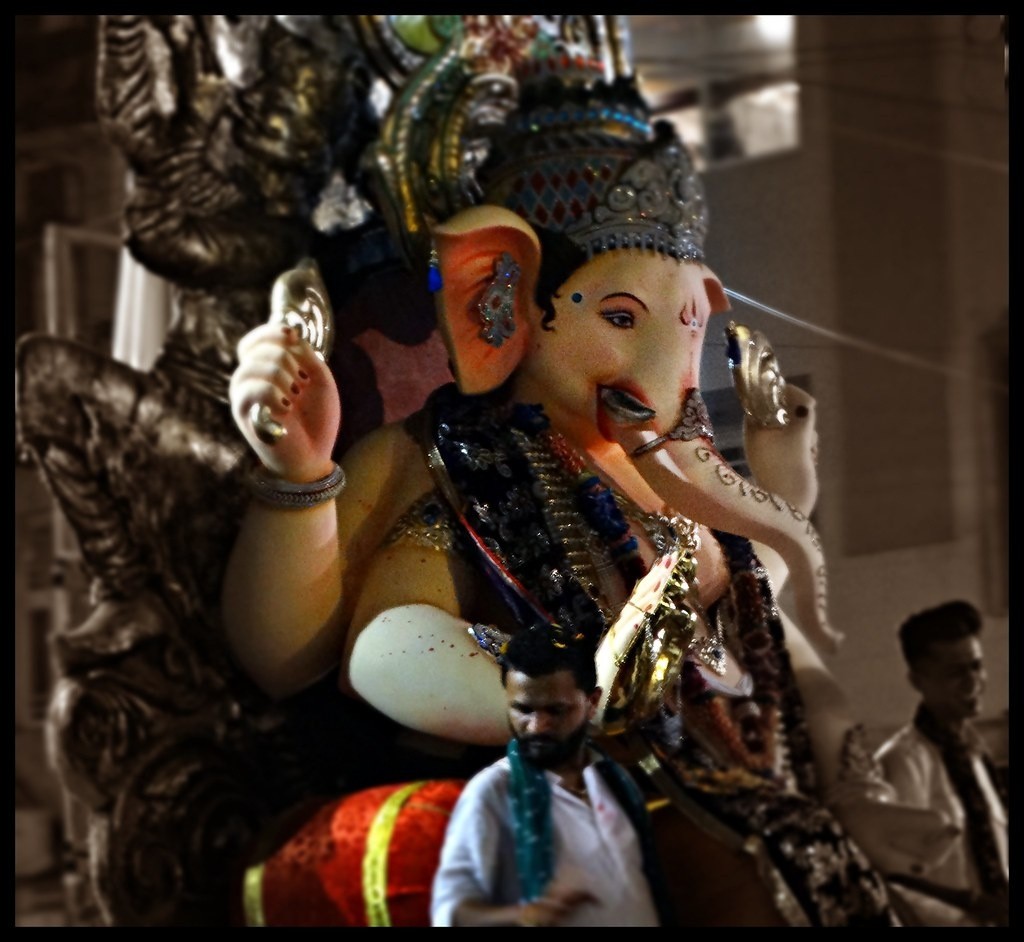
[429,624,658,929]
[865,598,1011,928]
